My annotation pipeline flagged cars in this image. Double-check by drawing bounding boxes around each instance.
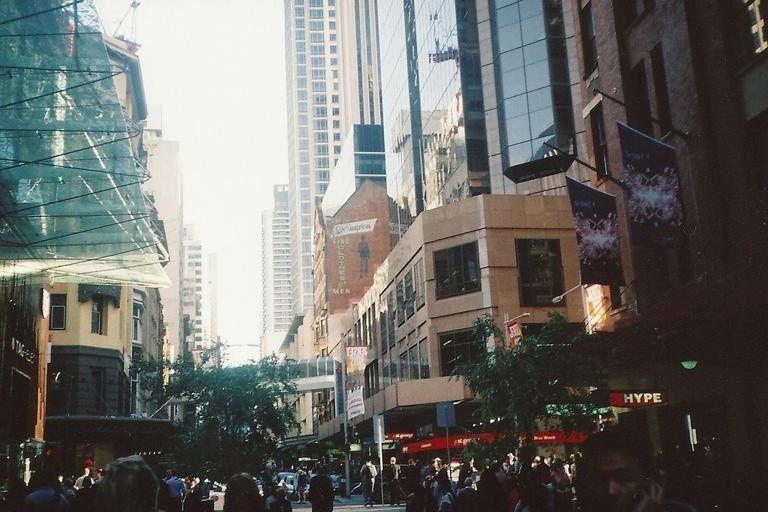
[190,479,226,510]
[277,472,295,499]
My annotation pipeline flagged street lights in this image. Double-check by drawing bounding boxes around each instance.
[504,312,530,352]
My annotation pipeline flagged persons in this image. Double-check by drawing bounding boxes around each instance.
[360,451,581,512]
[575,429,698,512]
[159,463,335,512]
[0,454,159,512]
